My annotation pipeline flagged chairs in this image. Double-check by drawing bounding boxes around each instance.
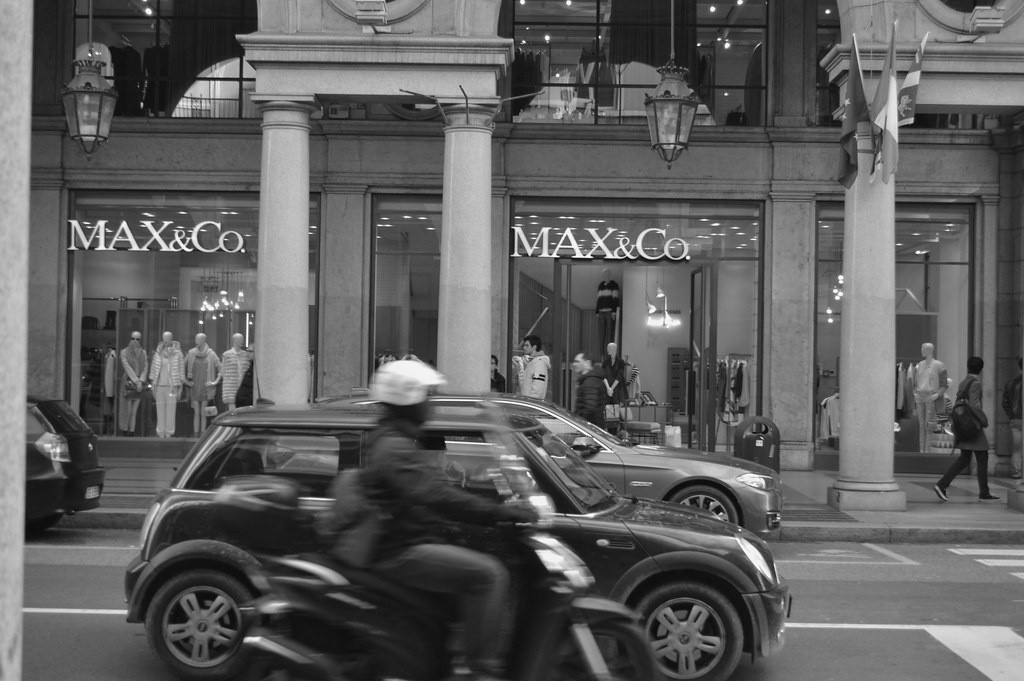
[621,406,661,445]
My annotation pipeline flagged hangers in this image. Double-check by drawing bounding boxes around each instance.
[114,34,131,48]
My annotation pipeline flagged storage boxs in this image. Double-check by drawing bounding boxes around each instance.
[211,476,301,548]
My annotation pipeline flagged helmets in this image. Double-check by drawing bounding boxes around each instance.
[370,360,448,408]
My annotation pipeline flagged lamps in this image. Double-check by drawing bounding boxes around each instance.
[644,265,674,327]
[969,6,1006,44]
[644,1,700,170]
[60,1,119,164]
[355,0,388,34]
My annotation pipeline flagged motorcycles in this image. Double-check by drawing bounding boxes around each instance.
[211,448,658,681]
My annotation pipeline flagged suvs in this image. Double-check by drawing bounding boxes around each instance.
[264,393,782,544]
[121,397,794,681]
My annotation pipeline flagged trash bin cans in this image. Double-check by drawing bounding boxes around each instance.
[734,416,780,474]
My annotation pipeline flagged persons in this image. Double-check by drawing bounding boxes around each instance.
[222,333,250,412]
[491,355,505,394]
[595,269,620,361]
[523,335,551,400]
[375,349,399,371]
[333,361,555,681]
[148,330,183,438]
[1002,358,1024,484]
[183,333,222,437]
[933,356,1001,502]
[235,344,254,408]
[602,343,640,405]
[914,343,948,454]
[573,351,608,431]
[400,350,425,362]
[118,332,148,437]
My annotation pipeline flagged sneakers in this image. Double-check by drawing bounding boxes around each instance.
[979,494,1000,502]
[934,485,949,501]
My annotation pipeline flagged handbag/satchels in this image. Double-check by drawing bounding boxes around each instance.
[603,396,620,420]
[203,406,218,417]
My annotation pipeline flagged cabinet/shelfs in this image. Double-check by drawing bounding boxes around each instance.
[623,401,674,449]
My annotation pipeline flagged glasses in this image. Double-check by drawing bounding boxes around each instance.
[131,338,140,341]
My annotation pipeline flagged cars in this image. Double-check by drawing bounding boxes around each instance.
[24,393,108,545]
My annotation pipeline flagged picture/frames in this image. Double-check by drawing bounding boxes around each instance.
[640,391,658,406]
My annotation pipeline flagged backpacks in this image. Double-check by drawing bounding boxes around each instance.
[951,379,981,439]
[1001,373,1023,420]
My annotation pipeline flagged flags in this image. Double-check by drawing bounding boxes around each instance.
[897,35,927,127]
[837,36,870,190]
[868,22,898,185]
